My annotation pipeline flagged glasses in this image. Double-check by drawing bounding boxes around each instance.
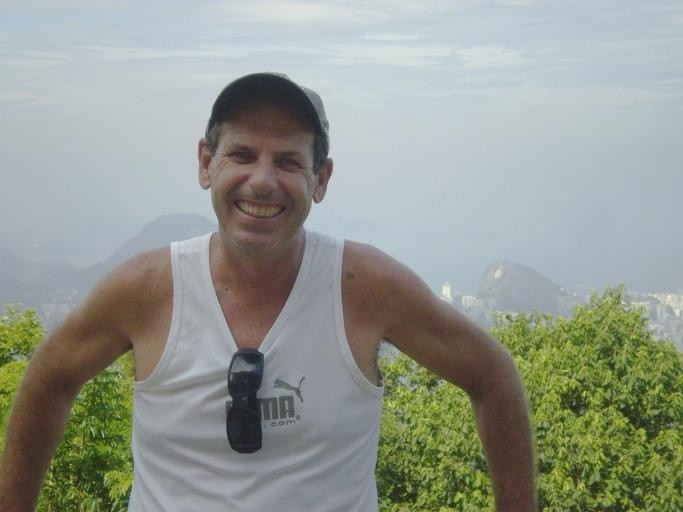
[227,348,263,453]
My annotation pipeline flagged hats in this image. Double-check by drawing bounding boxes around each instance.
[211,73,328,135]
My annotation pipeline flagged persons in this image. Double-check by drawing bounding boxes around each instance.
[3,72,537,512]
